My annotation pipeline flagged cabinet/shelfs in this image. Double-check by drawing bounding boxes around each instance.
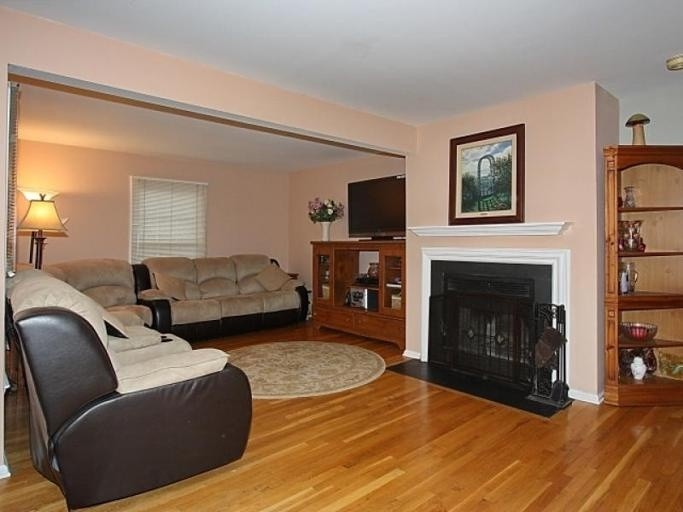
[310,240,407,349]
[602,144,683,407]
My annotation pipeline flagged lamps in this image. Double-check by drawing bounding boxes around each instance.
[15,186,60,264]
[17,199,70,271]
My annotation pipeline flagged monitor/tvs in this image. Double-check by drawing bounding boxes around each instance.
[346,172,405,242]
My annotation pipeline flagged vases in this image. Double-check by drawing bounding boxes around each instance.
[308,197,346,225]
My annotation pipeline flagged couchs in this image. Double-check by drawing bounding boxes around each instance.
[133,255,309,340]
[4,259,251,512]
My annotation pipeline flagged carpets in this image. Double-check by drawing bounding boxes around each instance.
[225,340,387,400]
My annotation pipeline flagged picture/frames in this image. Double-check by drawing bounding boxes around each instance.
[449,123,525,225]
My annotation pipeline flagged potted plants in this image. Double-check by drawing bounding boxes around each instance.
[321,223,331,240]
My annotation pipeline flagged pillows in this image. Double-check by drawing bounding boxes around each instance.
[151,273,184,302]
[238,277,264,295]
[82,285,137,308]
[201,277,239,298]
[254,263,292,292]
[183,281,200,301]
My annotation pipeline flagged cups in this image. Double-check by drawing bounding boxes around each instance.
[617,269,628,293]
[620,262,638,293]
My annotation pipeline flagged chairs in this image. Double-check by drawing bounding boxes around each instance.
[41,262,153,338]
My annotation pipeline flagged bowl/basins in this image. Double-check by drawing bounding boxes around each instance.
[618,321,657,342]
